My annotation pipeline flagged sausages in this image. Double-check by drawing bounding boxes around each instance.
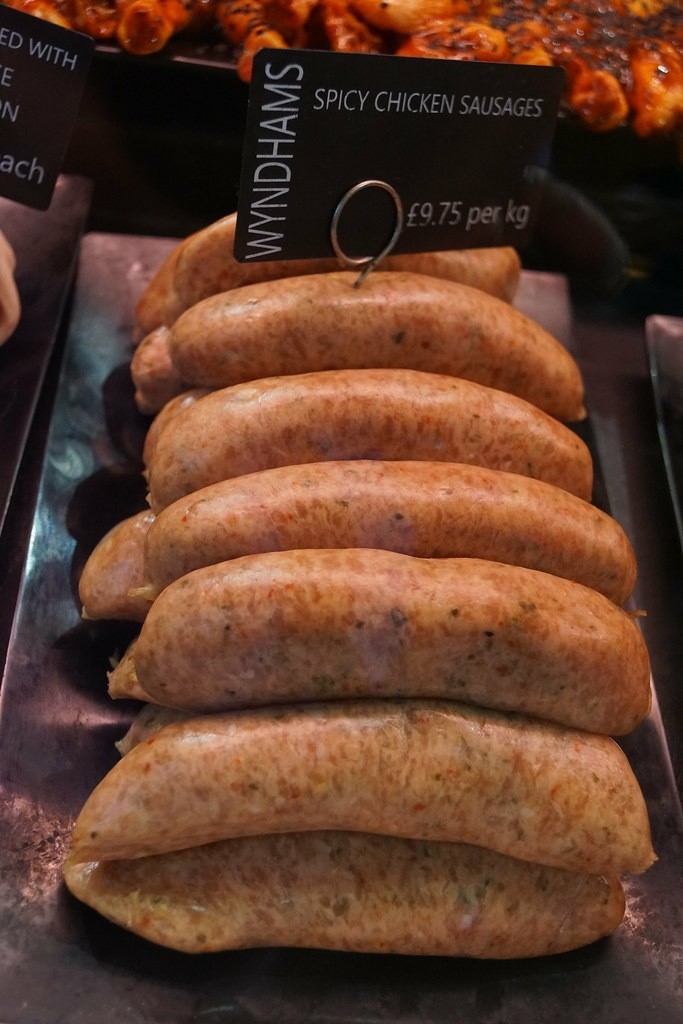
[61,206,658,958]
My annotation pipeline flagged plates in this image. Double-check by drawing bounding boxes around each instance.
[645,311,683,534]
[0,232,683,1024]
[1,169,97,532]
